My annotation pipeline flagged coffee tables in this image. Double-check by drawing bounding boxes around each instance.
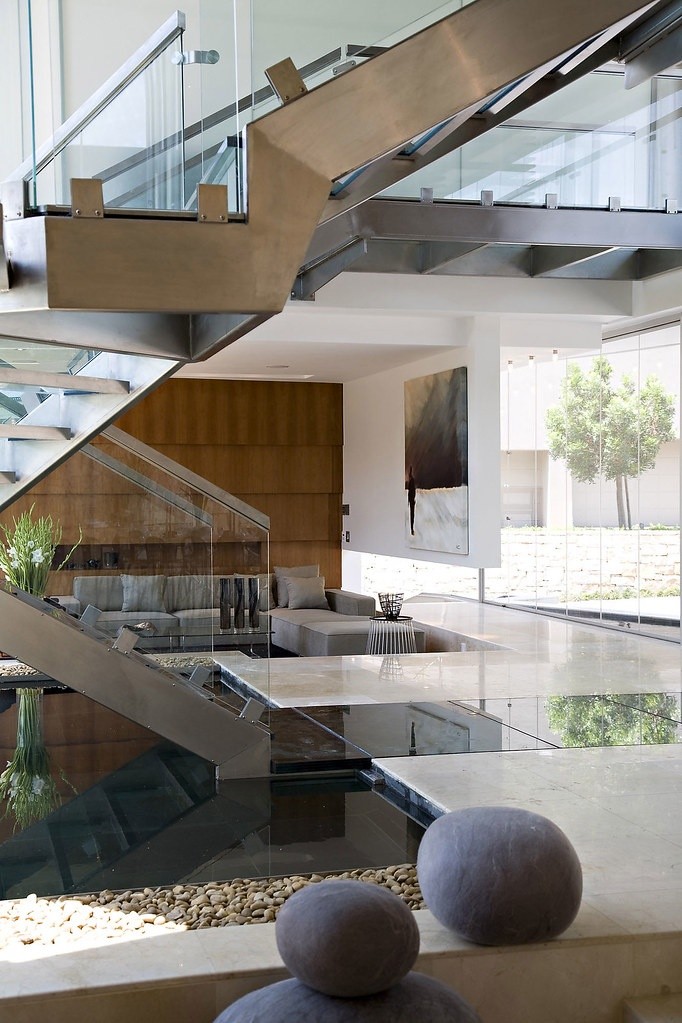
[97,625,274,659]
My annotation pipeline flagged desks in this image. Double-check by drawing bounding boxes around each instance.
[365,615,418,654]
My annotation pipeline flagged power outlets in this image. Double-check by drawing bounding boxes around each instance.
[346,531,350,542]
[342,531,346,542]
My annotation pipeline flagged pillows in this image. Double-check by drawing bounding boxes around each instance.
[232,573,275,611]
[120,573,167,613]
[273,564,319,609]
[284,576,331,610]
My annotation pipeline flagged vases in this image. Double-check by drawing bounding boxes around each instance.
[15,688,44,747]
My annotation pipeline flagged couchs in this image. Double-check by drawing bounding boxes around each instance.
[49,573,426,657]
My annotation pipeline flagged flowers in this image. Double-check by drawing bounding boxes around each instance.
[0,502,83,599]
[0,747,78,836]
